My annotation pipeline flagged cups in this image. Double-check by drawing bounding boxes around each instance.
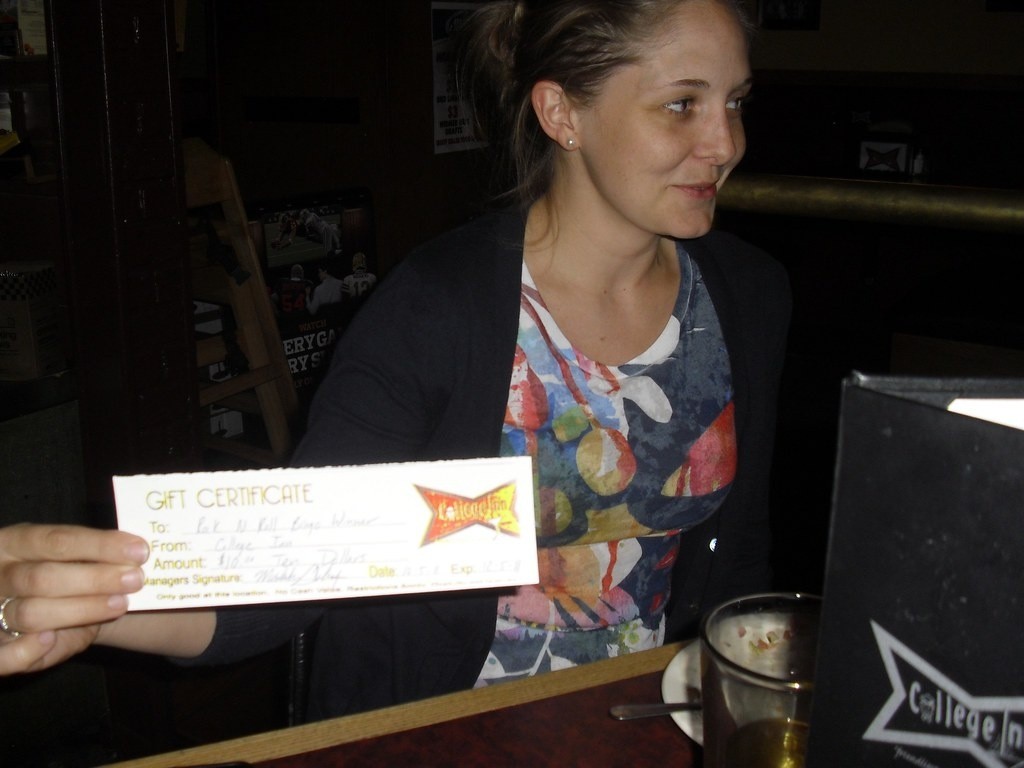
[701,591,823,768]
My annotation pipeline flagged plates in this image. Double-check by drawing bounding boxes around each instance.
[662,638,703,748]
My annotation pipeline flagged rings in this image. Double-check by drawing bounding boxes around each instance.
[0,597,19,636]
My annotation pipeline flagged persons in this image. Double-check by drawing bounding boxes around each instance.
[0,0,793,724]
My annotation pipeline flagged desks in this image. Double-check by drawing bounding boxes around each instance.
[98,637,708,768]
